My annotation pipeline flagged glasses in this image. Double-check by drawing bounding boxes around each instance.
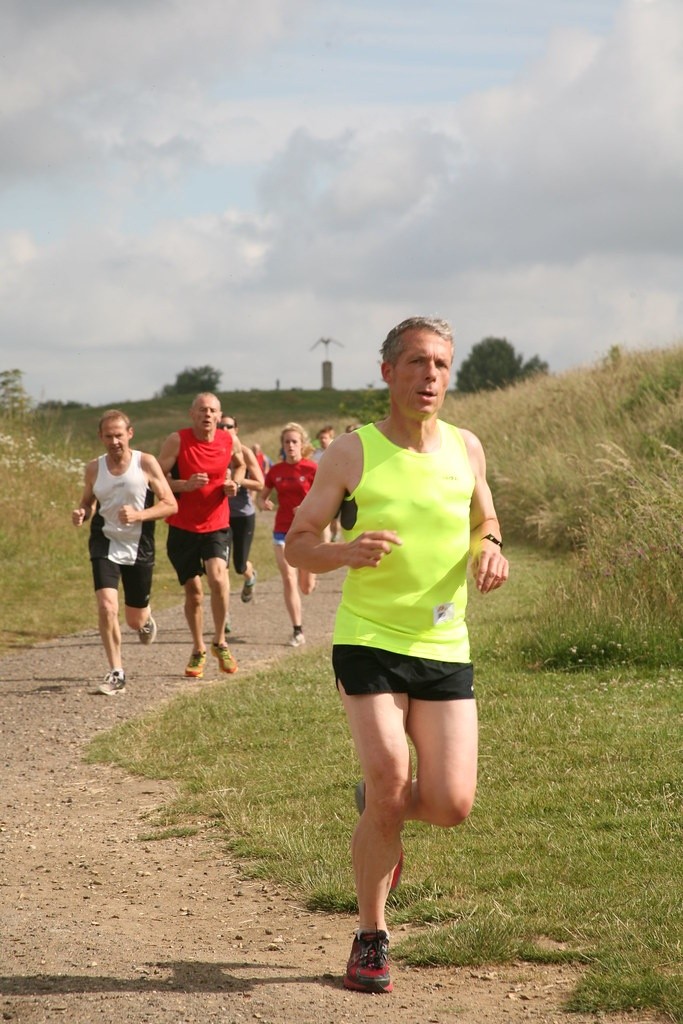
[218,424,234,429]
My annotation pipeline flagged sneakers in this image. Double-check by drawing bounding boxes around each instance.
[345,924,392,993]
[287,632,304,646]
[224,613,232,633]
[356,782,406,893]
[137,604,156,645]
[240,570,257,602]
[210,639,235,674]
[98,665,127,695]
[185,646,206,676]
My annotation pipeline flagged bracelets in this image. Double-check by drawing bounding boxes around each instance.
[234,481,241,493]
[480,535,503,550]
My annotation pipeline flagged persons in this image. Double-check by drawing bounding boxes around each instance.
[345,424,355,433]
[258,423,330,647]
[311,426,339,542]
[249,444,272,495]
[72,409,178,696]
[159,393,246,678]
[285,316,509,993]
[217,415,265,633]
